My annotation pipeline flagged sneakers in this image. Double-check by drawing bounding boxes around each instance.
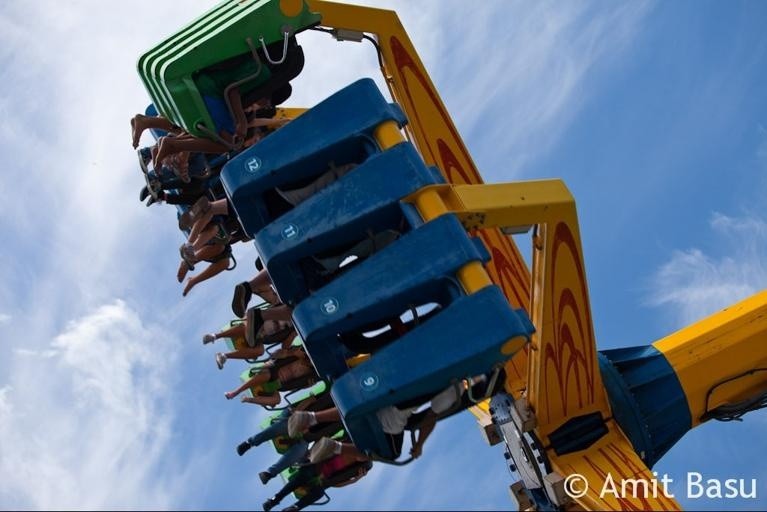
[138,147,210,270]
[204,282,263,371]
[237,411,336,511]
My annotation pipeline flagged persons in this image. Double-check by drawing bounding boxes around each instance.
[131,81,466,511]
